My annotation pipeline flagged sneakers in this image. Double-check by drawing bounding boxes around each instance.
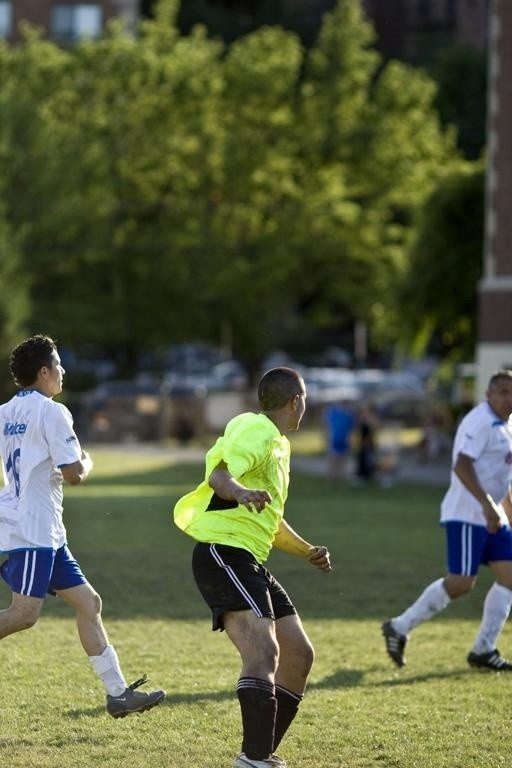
[231,750,289,768]
[105,686,166,720]
[381,619,407,669]
[466,648,511,671]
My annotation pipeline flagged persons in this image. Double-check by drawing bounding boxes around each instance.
[382,372,512,671]
[319,399,454,497]
[0,334,166,720]
[173,367,332,768]
